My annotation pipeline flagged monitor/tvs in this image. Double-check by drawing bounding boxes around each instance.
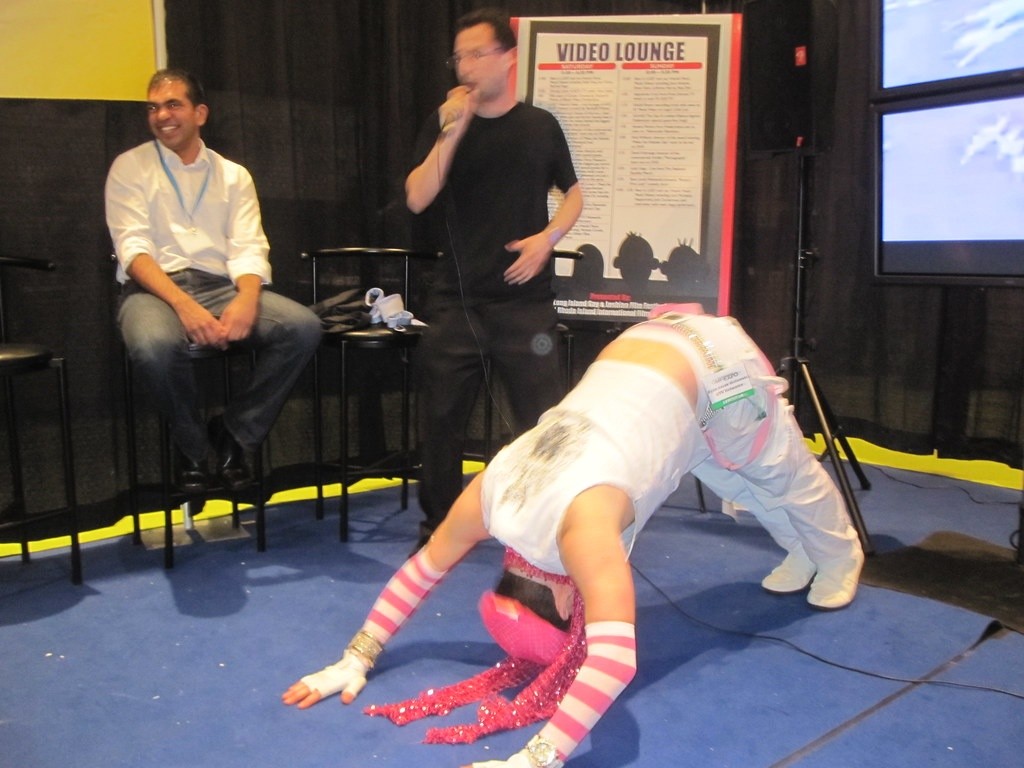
[868,82,1024,289]
[869,0,1024,100]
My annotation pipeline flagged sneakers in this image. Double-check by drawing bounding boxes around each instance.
[761,544,818,595]
[807,547,864,610]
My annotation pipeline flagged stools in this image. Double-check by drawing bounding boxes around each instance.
[0,256,81,587]
[298,247,422,542]
[110,254,265,567]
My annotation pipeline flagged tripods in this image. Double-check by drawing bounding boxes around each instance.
[774,153,876,560]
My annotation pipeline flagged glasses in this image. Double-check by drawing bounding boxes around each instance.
[449,47,505,65]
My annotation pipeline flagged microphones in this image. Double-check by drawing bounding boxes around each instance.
[443,83,475,128]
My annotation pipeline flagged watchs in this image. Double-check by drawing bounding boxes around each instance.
[526,735,563,768]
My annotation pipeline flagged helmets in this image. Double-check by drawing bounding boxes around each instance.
[478,588,570,666]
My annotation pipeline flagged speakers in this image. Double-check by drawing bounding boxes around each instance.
[742,0,841,153]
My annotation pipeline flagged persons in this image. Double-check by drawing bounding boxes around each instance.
[104,68,322,494]
[404,8,583,557]
[282,311,866,768]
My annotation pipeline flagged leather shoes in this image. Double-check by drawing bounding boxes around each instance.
[173,449,206,495]
[206,414,248,486]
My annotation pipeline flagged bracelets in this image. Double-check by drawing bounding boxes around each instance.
[349,631,383,666]
